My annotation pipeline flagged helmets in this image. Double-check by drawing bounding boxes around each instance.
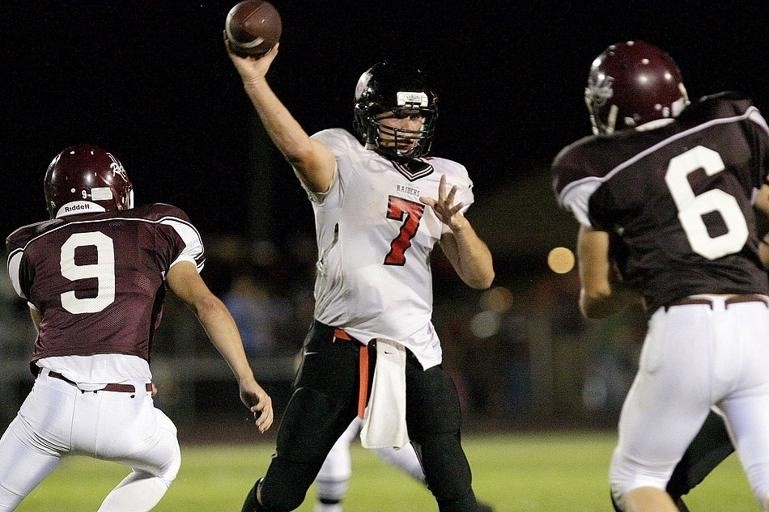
[353,57,438,140]
[584,40,692,135]
[43,142,135,219]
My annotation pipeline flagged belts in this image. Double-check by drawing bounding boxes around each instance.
[668,293,769,310]
[333,328,356,340]
[36,365,152,394]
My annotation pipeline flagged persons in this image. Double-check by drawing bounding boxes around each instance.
[0,140,274,512]
[316,405,425,512]
[551,43,768,512]
[222,29,495,511]
[611,408,736,511]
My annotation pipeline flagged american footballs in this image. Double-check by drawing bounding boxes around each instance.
[226,1,282,58]
